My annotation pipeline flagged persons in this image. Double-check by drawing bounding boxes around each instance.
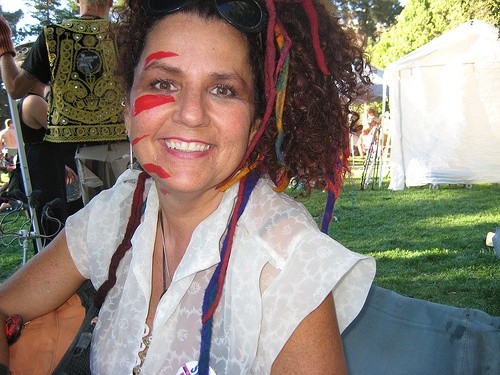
[1,139,84,222]
[1,0,130,256]
[339,107,390,158]
[9,263,499,375]
[0,118,17,172]
[12,52,80,257]
[0,0,379,375]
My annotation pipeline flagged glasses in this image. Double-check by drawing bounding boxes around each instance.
[147,0,268,46]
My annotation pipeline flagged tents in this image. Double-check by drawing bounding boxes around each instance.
[329,55,389,128]
[381,18,500,191]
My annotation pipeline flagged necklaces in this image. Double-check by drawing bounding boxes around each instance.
[158,208,169,302]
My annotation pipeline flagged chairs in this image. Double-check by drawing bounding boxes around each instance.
[340,282,500,375]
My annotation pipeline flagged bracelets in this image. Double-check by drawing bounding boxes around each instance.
[0,308,22,345]
